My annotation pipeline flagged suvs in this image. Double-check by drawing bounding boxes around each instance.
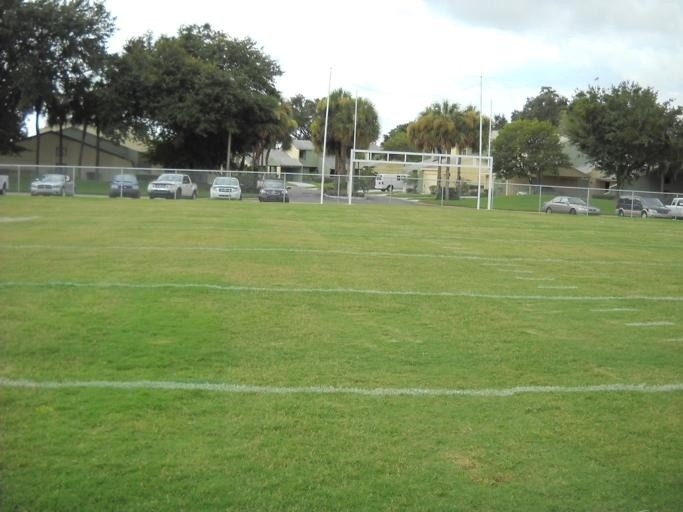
[615,194,672,219]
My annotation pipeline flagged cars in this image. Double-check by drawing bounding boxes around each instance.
[28,173,79,196]
[106,171,141,200]
[257,178,293,203]
[207,175,244,200]
[542,196,601,216]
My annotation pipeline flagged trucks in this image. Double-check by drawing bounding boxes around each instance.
[374,173,415,192]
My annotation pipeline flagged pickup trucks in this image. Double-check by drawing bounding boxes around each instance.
[665,197,683,221]
[145,171,200,199]
[1,175,10,193]
